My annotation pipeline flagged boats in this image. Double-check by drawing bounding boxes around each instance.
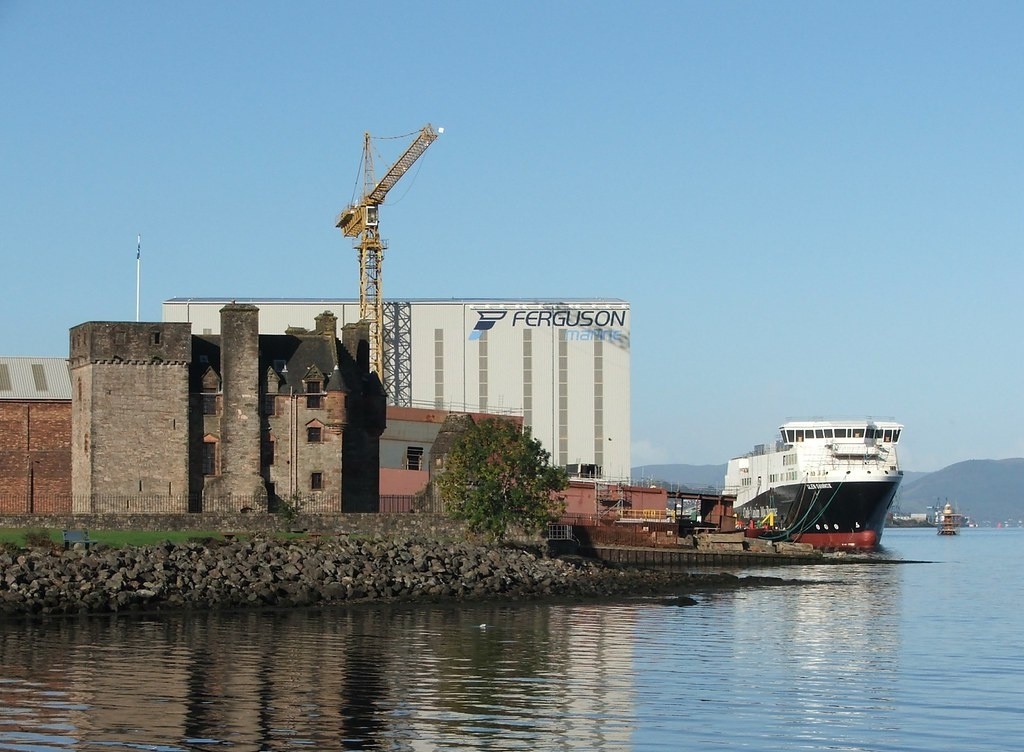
[934,496,957,535]
[738,527,766,538]
[719,420,904,551]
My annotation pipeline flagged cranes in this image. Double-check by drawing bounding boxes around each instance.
[333,121,440,408]
